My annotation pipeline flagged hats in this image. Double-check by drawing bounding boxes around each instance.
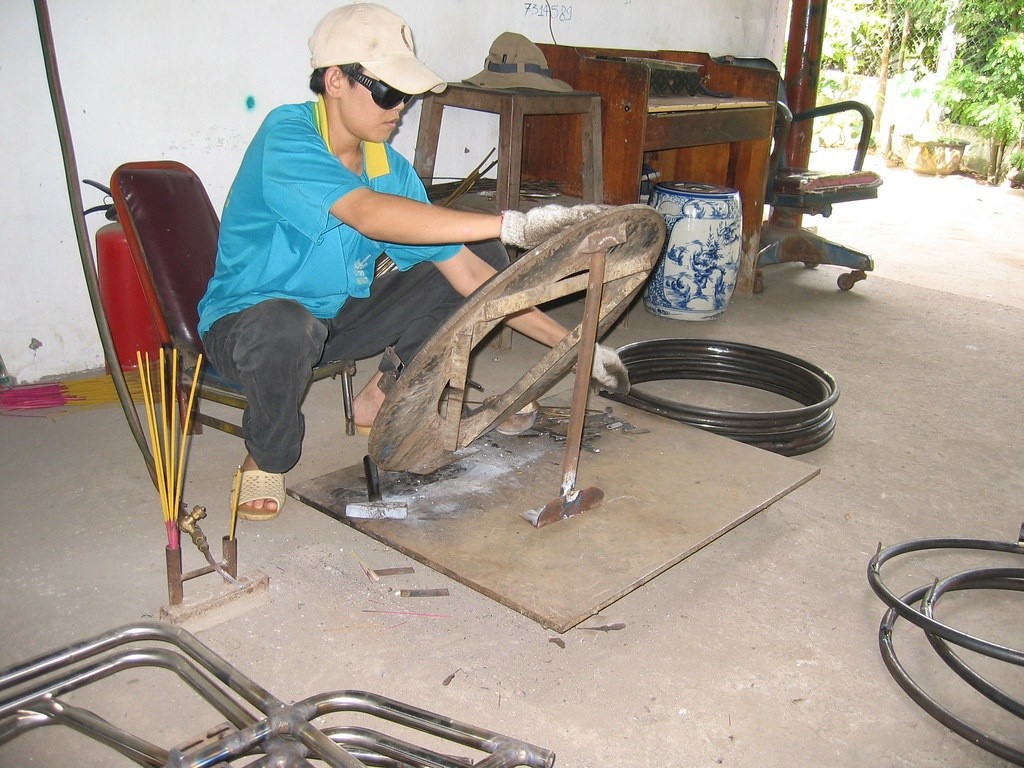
[308,2,447,94]
[462,32,573,93]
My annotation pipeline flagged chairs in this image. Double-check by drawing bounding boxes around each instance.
[109,160,356,449]
[714,55,883,295]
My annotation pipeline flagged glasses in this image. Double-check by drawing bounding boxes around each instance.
[348,69,416,110]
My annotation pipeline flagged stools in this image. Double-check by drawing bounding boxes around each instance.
[642,180,743,322]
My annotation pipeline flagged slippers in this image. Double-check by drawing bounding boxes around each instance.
[356,425,372,437]
[230,470,286,521]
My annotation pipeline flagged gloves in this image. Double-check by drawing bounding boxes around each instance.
[571,342,631,395]
[500,204,615,249]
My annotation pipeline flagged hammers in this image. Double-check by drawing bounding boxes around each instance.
[345,455,409,520]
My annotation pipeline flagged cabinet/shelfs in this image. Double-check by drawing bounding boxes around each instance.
[528,42,783,299]
[412,81,605,350]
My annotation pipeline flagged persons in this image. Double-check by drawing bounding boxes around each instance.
[198,5,631,520]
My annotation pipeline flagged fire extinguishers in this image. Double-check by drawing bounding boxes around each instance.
[81,178,175,376]
